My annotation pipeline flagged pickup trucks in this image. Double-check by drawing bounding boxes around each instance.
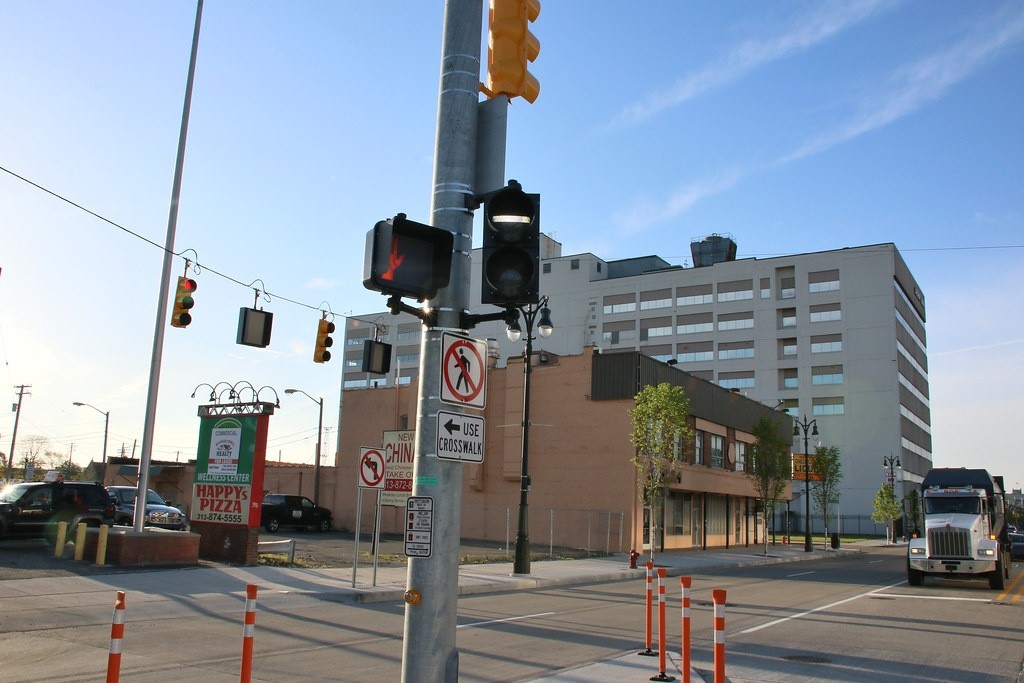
[262,493,333,534]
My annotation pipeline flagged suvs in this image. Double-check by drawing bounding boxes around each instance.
[104,486,186,531]
[0,470,116,545]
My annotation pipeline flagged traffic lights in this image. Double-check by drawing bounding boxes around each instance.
[171,275,197,328]
[314,319,336,364]
[236,308,274,349]
[362,213,455,302]
[362,340,392,375]
[482,187,541,306]
[487,0,541,104]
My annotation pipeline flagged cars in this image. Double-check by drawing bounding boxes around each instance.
[1006,533,1024,560]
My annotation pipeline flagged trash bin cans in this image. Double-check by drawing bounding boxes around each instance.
[831,533,840,549]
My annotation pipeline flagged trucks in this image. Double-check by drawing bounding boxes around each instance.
[907,467,1012,591]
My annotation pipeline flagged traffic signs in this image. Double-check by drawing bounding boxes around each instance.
[437,410,485,464]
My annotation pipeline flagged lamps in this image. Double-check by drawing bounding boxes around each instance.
[191,381,280,412]
[730,388,740,392]
[667,359,678,365]
[781,409,789,412]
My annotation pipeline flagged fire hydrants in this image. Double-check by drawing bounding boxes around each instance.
[628,549,640,569]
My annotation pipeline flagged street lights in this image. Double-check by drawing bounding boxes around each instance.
[504,295,553,574]
[793,414,819,552]
[284,388,323,506]
[73,402,109,485]
[882,453,902,543]
